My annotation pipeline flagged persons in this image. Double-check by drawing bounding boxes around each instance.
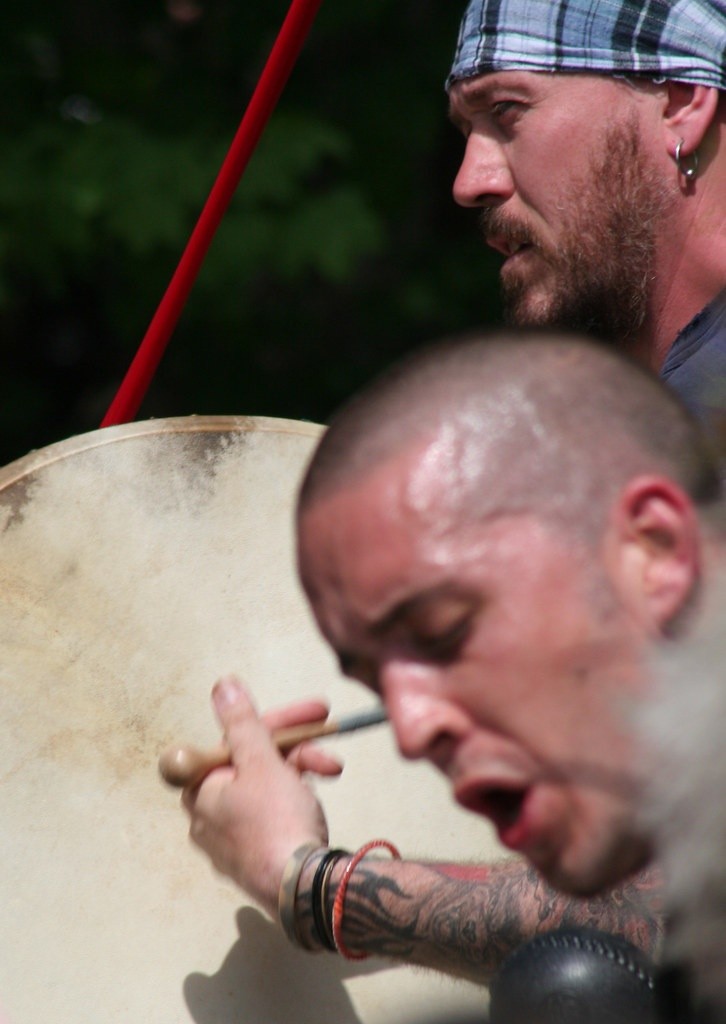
[181,330,725,1024]
[443,1,726,501]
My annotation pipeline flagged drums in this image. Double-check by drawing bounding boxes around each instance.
[0,413,538,1024]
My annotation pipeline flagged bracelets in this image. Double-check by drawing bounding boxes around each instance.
[278,840,402,963]
